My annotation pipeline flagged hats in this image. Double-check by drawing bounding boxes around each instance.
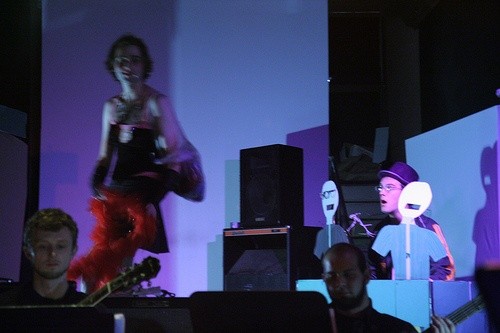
[377,162,419,186]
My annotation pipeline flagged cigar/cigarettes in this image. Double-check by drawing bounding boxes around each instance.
[131,73,140,78]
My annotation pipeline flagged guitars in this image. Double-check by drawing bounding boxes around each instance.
[422,294,485,333]
[78,256,161,306]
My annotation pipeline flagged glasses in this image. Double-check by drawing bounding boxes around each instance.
[375,184,403,191]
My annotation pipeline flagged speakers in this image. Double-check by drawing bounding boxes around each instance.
[240,144,304,232]
[223,225,324,291]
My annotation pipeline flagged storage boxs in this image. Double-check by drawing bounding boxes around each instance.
[223,226,322,290]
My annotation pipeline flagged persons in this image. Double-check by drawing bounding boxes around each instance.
[86,33,206,295]
[1,209,106,313]
[319,242,458,333]
[367,163,454,280]
